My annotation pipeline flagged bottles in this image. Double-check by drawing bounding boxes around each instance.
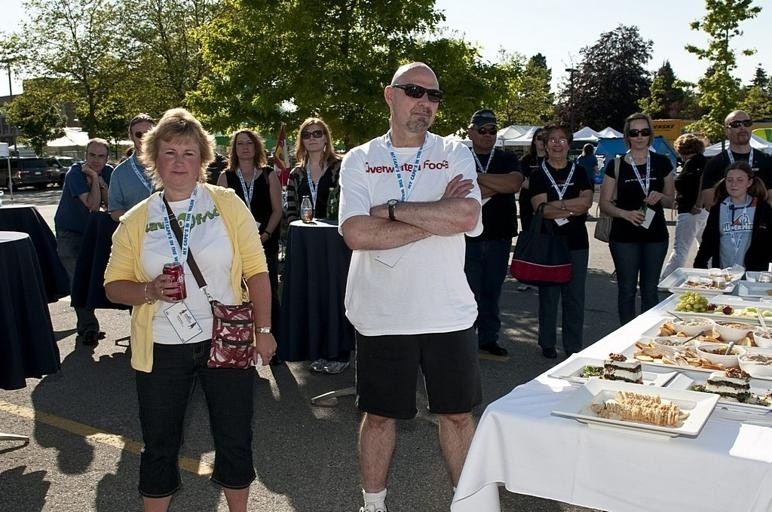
[636,201,647,225]
[300,196,313,223]
[326,188,338,220]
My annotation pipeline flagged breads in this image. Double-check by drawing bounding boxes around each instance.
[659,324,687,338]
[634,344,697,369]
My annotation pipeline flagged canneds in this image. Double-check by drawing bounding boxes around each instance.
[162,262,187,303]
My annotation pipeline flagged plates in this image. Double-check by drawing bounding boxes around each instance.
[614,267,772,381]
[549,356,679,387]
[682,381,771,416]
[551,378,721,437]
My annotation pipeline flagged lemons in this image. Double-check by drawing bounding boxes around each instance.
[746,307,756,312]
[761,310,772,316]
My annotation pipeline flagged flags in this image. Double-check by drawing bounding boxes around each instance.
[271,122,292,181]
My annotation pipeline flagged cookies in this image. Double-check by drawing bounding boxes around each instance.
[590,391,688,426]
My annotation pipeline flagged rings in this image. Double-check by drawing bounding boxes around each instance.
[160,289,164,295]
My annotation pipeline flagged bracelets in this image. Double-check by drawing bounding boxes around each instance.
[561,199,566,211]
[254,326,272,333]
[143,281,153,304]
[264,230,273,236]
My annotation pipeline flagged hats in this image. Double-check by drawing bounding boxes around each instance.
[469,108,498,128]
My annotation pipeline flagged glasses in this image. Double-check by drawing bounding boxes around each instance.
[547,138,568,146]
[300,130,323,139]
[628,128,651,137]
[730,119,753,128]
[393,83,443,102]
[477,126,498,136]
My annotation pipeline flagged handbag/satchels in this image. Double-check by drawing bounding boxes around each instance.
[509,225,574,286]
[206,299,256,369]
[594,201,617,243]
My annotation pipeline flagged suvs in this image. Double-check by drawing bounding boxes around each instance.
[0,155,51,194]
[42,155,73,186]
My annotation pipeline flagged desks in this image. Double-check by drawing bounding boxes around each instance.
[287,219,361,409]
[2,206,72,304]
[0,229,64,448]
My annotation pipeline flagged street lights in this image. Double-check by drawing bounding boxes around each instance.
[566,67,579,132]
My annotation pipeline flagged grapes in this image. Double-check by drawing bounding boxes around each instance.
[676,291,707,312]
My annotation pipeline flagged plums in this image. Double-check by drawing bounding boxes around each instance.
[723,305,731,314]
[707,304,717,310]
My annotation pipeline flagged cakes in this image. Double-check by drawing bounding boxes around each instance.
[703,368,752,402]
[603,352,642,382]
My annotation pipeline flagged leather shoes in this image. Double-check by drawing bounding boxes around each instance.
[477,337,508,355]
[542,345,558,360]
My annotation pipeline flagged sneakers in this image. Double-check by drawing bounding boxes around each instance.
[309,358,349,374]
[78,327,106,346]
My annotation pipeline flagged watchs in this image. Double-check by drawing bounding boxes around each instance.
[569,211,572,216]
[386,199,399,220]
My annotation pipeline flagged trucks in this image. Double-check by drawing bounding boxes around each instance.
[648,118,699,168]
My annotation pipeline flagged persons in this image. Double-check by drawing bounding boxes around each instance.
[594,112,674,327]
[103,106,278,511]
[105,112,160,224]
[573,142,598,220]
[467,109,522,357]
[215,129,282,367]
[519,127,546,232]
[53,137,116,345]
[694,161,771,276]
[659,133,708,279]
[697,109,772,213]
[523,120,594,359]
[282,116,352,229]
[338,61,484,511]
